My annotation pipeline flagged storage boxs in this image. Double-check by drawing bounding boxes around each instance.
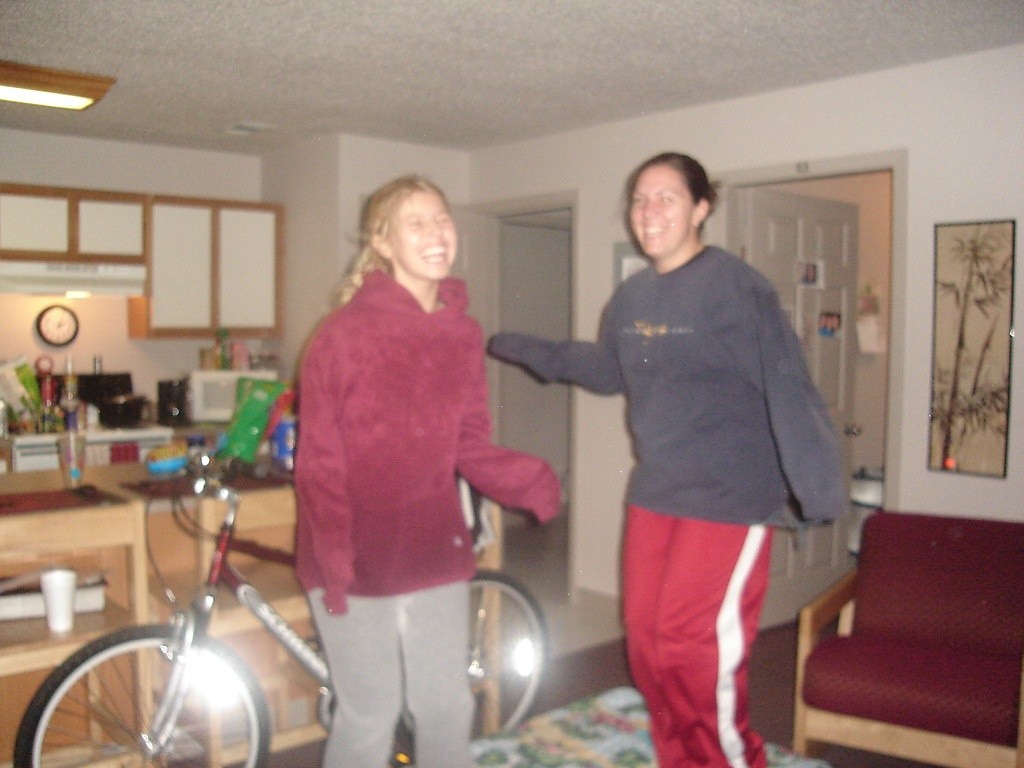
[0,575,108,620]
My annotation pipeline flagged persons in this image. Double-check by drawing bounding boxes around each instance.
[485,151,849,768]
[292,175,562,768]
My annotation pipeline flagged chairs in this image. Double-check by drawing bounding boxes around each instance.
[793,513,1024,768]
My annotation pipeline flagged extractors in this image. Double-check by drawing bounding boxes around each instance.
[0,259,146,300]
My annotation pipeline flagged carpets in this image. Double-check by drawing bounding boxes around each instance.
[468,686,831,768]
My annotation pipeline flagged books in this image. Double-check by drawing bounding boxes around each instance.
[0,571,107,620]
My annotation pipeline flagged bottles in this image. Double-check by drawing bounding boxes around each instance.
[216,331,233,369]
[56,402,87,489]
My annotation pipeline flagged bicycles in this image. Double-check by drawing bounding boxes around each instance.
[9,448,551,768]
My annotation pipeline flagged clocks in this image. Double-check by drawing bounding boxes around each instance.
[37,305,79,346]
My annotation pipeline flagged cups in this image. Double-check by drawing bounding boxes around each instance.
[40,569,75,632]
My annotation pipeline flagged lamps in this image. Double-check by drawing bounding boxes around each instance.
[0,60,116,112]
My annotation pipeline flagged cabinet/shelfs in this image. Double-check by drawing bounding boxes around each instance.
[128,195,286,340]
[1,182,149,264]
[0,464,501,768]
